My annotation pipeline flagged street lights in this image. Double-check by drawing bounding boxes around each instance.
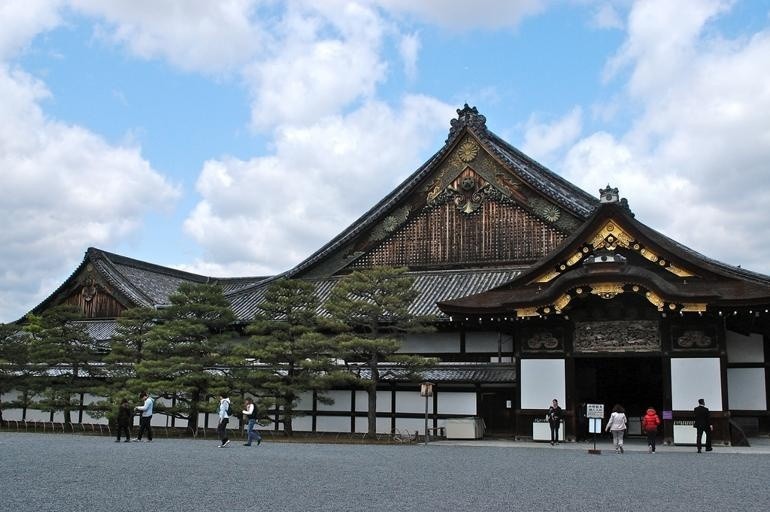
[419,381,438,446]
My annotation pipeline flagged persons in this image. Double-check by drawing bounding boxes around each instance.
[115,398,130,442]
[604,404,628,454]
[694,399,714,453]
[642,406,661,454]
[546,398,562,446]
[216,392,231,447]
[133,393,153,442]
[242,397,262,446]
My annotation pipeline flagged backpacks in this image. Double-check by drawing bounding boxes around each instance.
[222,400,232,416]
